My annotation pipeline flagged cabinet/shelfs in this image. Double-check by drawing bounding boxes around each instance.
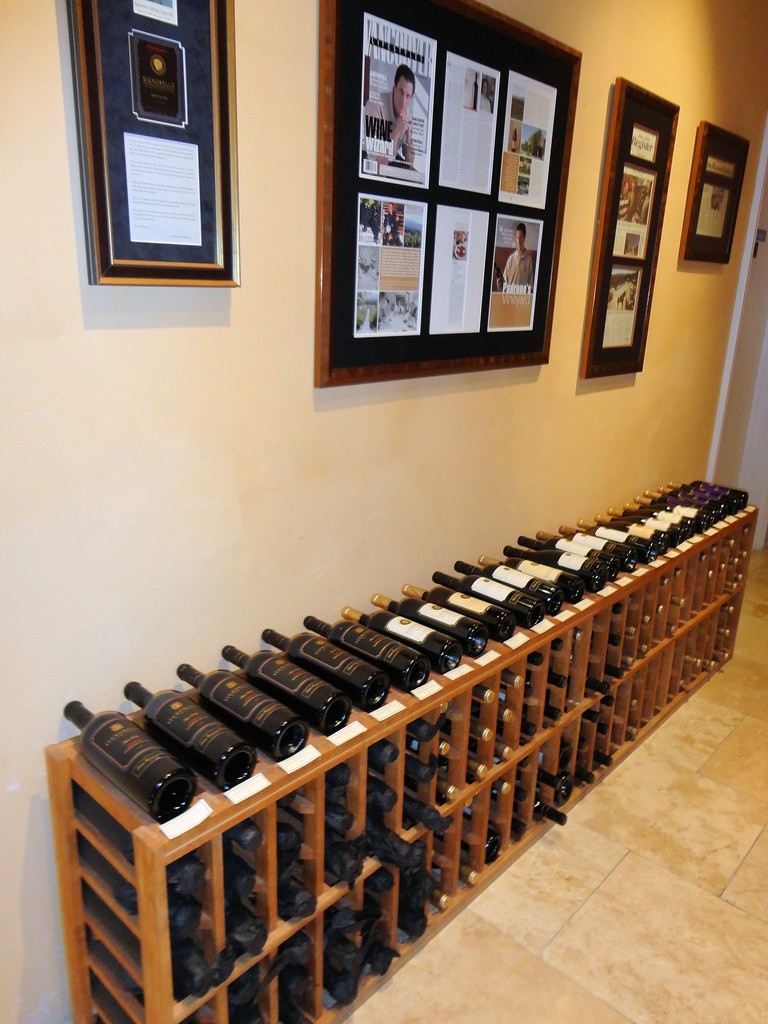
[45,506,760,1024]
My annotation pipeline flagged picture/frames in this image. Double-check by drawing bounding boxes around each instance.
[314,0,584,387]
[67,0,242,288]
[579,76,681,381]
[678,121,750,264]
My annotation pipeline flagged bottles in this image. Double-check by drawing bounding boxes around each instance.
[54,479,749,1024]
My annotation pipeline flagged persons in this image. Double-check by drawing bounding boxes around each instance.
[360,199,402,246]
[476,78,490,113]
[365,64,416,169]
[618,182,653,225]
[379,299,418,332]
[496,223,534,294]
